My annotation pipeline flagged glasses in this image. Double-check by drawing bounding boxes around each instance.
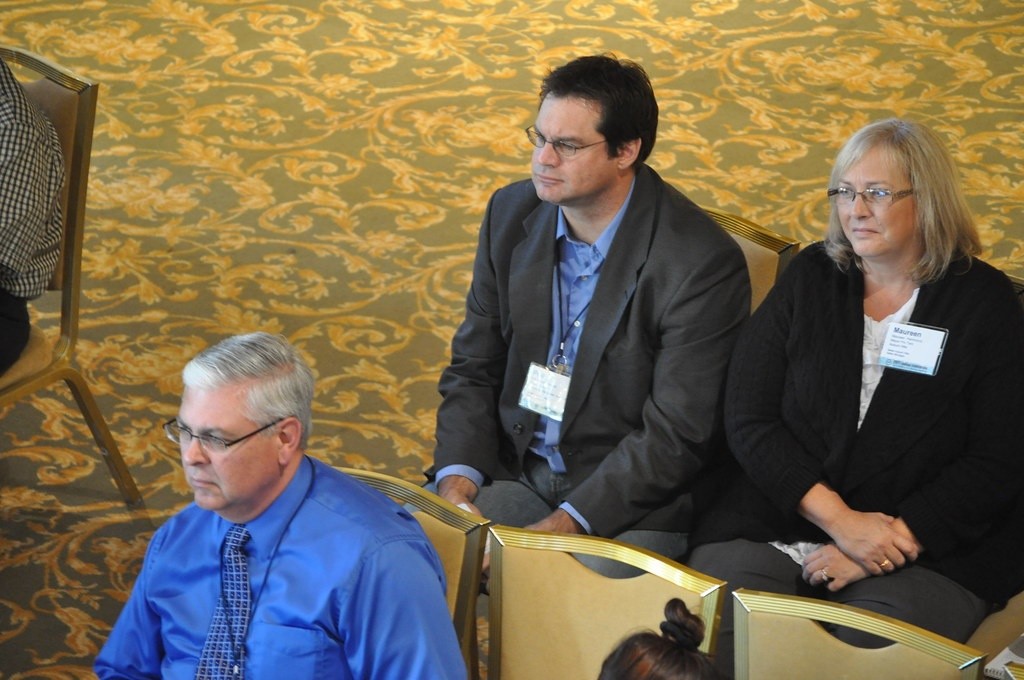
[163,418,283,454]
[525,125,607,158]
[828,187,913,208]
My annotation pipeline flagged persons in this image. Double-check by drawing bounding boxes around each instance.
[596,596,734,680]
[91,329,475,680]
[400,54,752,619]
[682,119,1024,680]
[0,61,67,376]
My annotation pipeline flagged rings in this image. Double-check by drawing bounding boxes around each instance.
[879,559,890,567]
[819,568,828,583]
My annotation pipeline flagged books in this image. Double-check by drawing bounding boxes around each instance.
[982,631,1024,680]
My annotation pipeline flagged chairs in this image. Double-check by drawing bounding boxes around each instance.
[730,587,988,680]
[0,42,147,516]
[486,522,727,680]
[333,465,492,680]
[696,205,803,316]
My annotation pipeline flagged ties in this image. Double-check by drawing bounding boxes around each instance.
[193,524,251,680]
